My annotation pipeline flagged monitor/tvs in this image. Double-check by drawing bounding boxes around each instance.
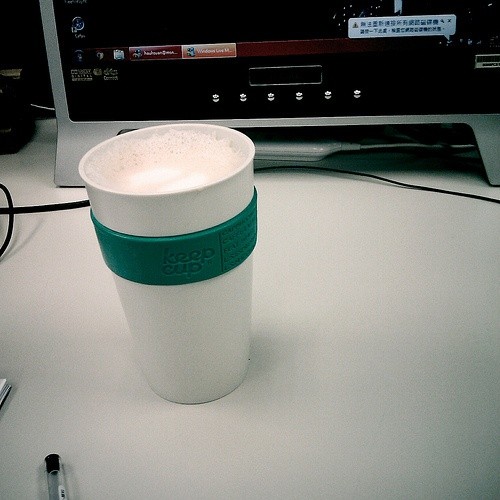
[36,0,500,126]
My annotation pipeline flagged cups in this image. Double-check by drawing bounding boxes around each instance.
[79,126,258,405]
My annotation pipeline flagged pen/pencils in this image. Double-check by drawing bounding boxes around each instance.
[44,453,69,500]
